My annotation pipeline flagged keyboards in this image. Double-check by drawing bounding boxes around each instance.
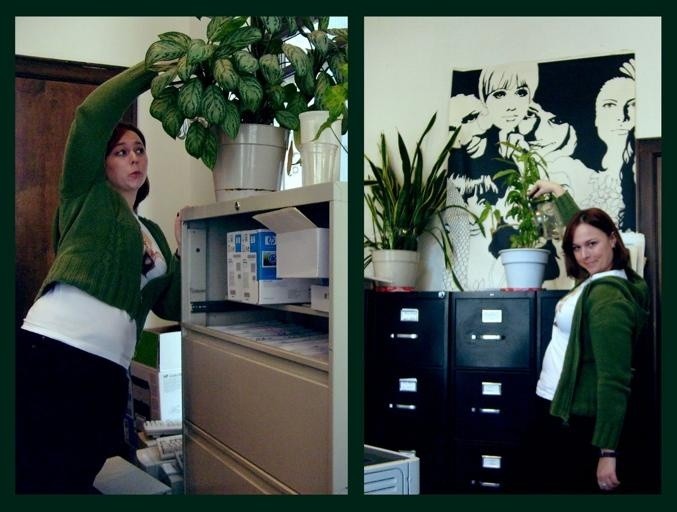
[142,419,181,436]
[157,434,184,460]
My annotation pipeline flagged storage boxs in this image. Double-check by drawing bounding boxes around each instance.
[224,210,327,306]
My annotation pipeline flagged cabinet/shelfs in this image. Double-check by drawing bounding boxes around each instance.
[363,292,573,496]
[180,330,332,497]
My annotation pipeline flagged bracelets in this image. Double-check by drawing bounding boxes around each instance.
[598,451,618,459]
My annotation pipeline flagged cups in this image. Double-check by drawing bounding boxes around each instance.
[302,142,339,187]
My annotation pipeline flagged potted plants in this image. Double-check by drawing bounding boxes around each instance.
[147,4,349,203]
[469,137,560,292]
[360,111,487,292]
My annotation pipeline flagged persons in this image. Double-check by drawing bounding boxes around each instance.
[506,179,654,496]
[12,56,179,497]
